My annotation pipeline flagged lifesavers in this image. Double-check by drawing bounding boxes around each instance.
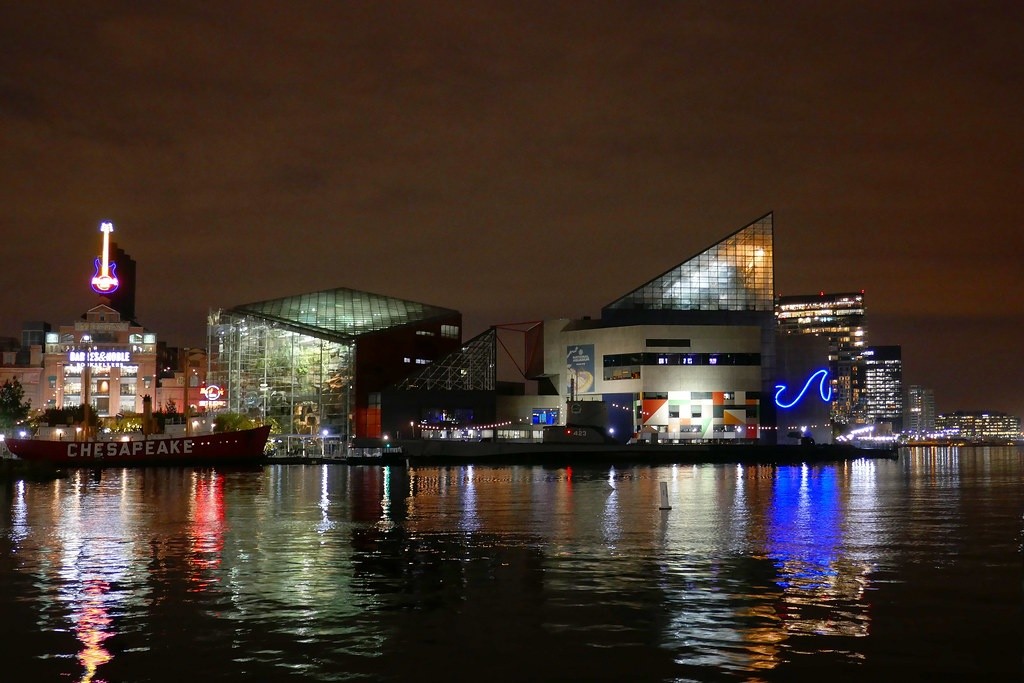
[571,403,582,414]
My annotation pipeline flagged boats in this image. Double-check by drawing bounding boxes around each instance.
[4,423,273,466]
[345,438,412,467]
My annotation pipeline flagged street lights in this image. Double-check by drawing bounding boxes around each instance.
[82,335,90,366]
[411,422,414,438]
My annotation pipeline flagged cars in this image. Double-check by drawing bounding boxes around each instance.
[300,439,321,455]
[1007,440,1024,446]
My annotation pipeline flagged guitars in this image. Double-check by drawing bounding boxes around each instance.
[90,221,120,294]
